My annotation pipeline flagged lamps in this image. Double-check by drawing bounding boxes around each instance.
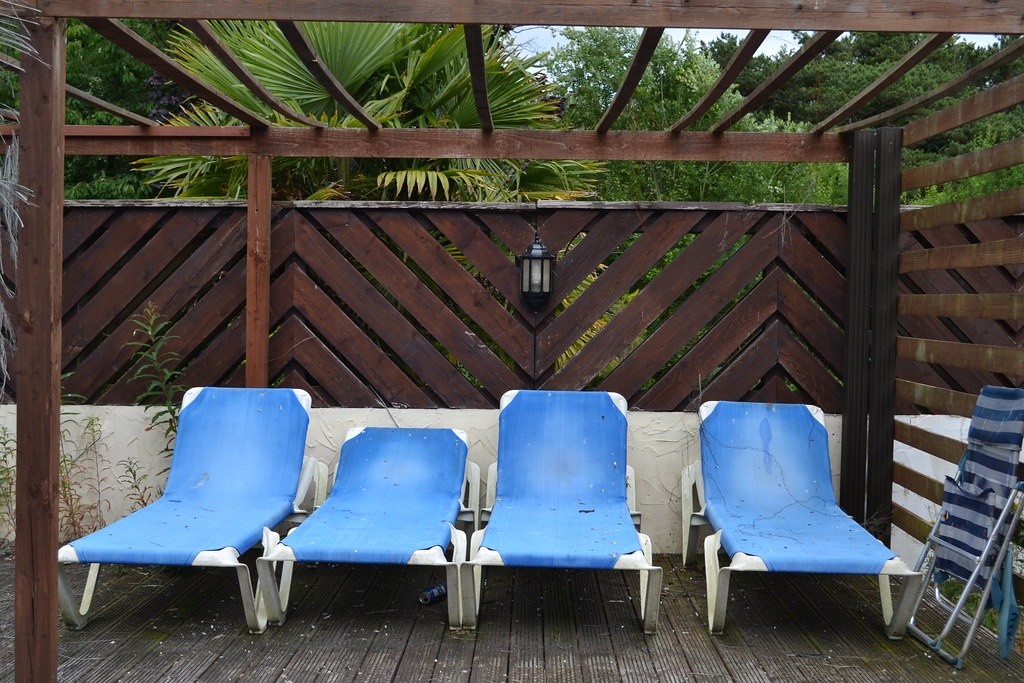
[519,228,553,317]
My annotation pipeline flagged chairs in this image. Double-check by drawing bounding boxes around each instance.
[681,398,923,641]
[907,384,1024,672]
[255,425,468,632]
[463,389,663,637]
[60,388,312,636]
